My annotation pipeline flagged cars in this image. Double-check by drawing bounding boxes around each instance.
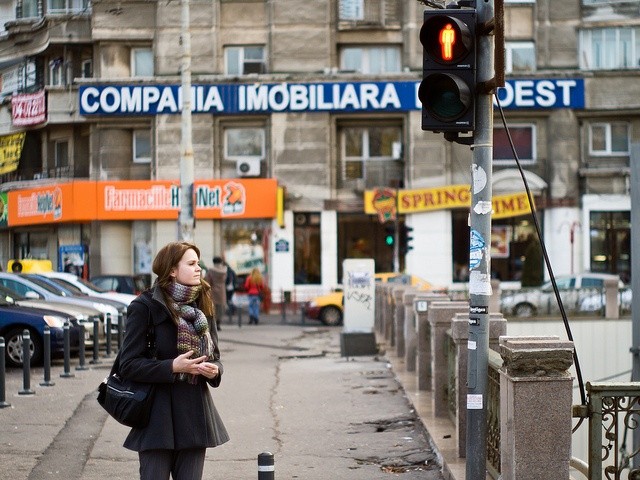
[17,274,127,317]
[500,273,632,318]
[308,272,433,325]
[0,273,119,335]
[42,273,138,306]
[1,298,83,368]
[0,284,107,354]
[89,276,151,295]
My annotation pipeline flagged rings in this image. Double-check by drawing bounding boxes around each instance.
[212,370,215,374]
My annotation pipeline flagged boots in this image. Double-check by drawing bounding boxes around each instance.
[248,316,254,324]
[254,318,258,325]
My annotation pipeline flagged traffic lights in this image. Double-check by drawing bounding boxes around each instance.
[418,9,477,131]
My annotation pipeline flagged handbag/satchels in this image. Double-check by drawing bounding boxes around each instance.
[258,292,264,302]
[97,373,157,429]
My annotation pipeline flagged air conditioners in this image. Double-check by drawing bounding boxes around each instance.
[339,0,365,21]
[236,155,262,178]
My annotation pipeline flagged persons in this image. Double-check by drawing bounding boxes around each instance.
[204,257,228,331]
[244,269,264,324]
[222,262,235,316]
[96,242,231,480]
